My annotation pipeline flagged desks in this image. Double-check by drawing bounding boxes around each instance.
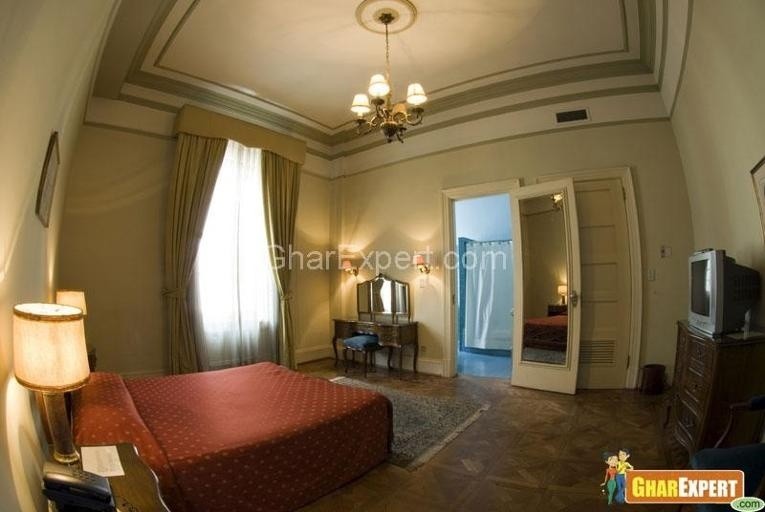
[329,317,420,379]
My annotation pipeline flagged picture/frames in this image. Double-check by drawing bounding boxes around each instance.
[34,129,62,231]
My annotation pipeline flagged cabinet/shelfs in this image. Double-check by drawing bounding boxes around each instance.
[663,317,765,459]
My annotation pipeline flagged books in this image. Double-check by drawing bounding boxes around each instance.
[78,445,126,478]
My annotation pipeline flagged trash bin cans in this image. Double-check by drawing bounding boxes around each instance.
[640,364,665,395]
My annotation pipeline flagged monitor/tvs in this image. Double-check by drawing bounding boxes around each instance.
[688,249,760,338]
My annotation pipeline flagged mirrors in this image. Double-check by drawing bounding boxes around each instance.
[518,188,571,370]
[355,273,411,323]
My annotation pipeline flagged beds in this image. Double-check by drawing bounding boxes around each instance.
[31,358,395,512]
[522,312,567,352]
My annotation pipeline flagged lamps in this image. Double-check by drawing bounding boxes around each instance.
[9,300,93,465]
[556,281,568,303]
[412,254,432,275]
[549,192,566,209]
[53,286,89,318]
[349,1,432,149]
[342,260,359,276]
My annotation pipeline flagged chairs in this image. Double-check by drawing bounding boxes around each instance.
[341,333,397,377]
[687,393,765,512]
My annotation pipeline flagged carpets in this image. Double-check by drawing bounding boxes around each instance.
[522,347,567,366]
[325,375,492,474]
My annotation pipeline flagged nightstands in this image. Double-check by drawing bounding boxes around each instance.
[546,303,567,316]
[87,341,98,373]
[46,439,172,512]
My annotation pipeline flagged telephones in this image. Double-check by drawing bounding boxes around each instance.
[41,461,116,512]
